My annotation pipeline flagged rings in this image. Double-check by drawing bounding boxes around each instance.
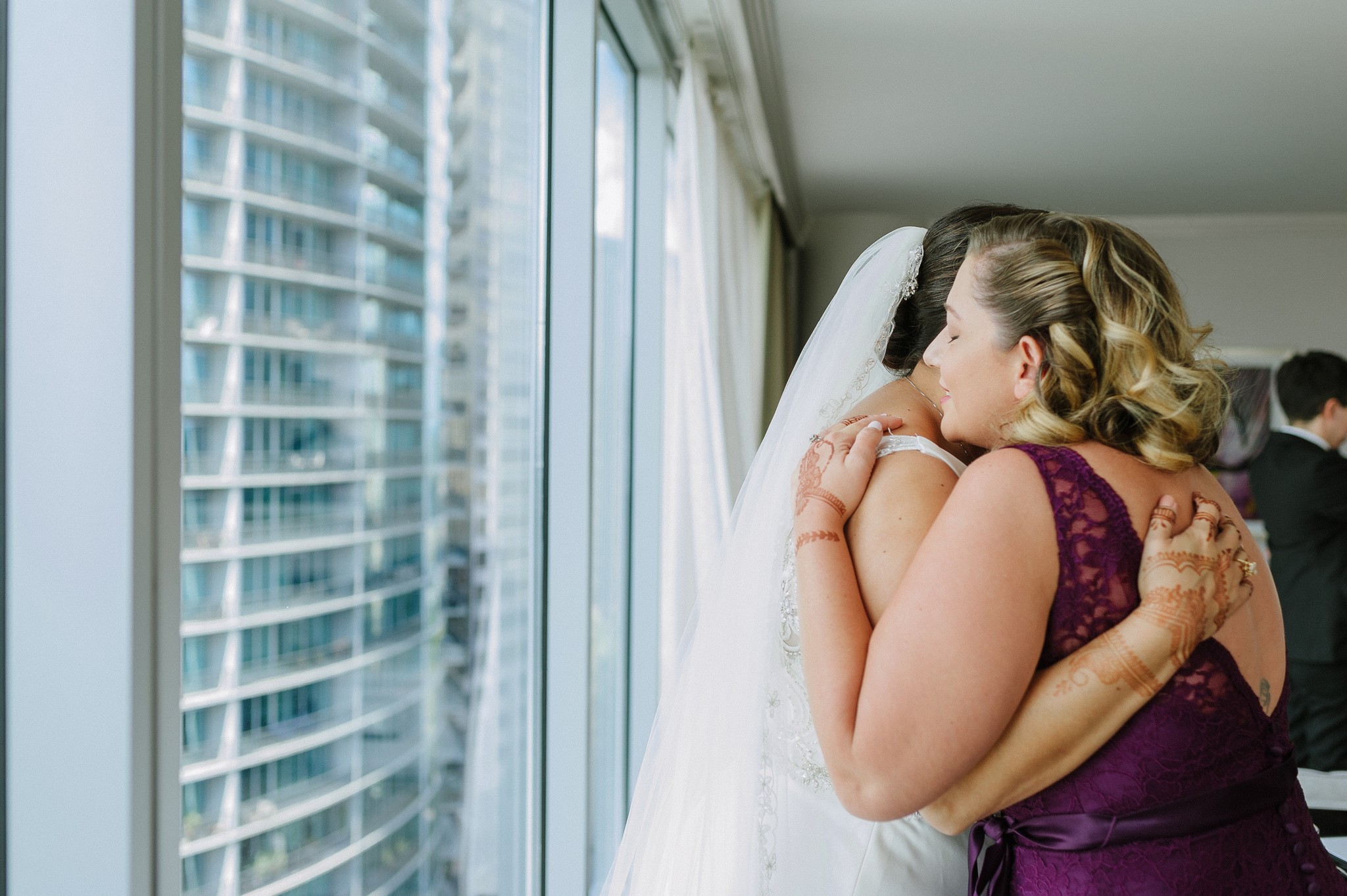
[808,434,822,445]
[1236,559,1257,578]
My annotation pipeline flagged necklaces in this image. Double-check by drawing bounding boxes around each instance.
[901,374,975,463]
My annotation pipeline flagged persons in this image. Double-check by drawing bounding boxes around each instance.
[1247,349,1346,840]
[791,211,1347,895]
[601,205,1254,895]
